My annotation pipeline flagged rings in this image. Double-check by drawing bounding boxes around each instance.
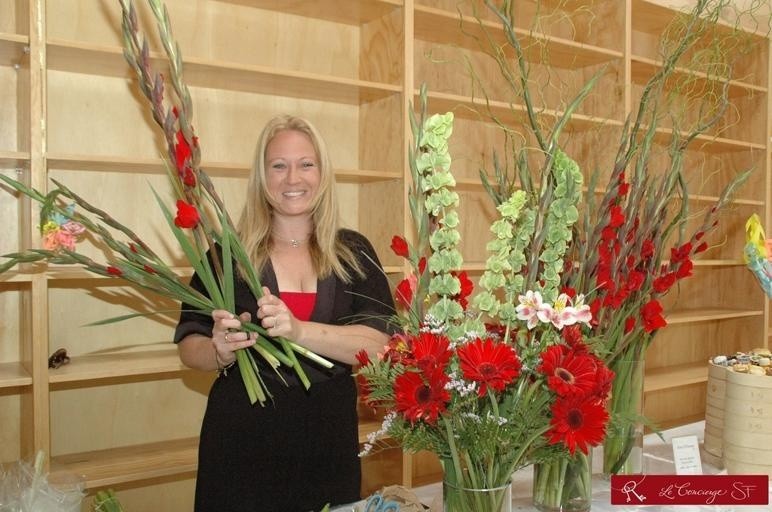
[225,332,230,341]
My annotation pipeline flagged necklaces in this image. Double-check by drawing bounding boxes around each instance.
[265,234,312,250]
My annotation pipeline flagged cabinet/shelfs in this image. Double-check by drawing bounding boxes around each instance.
[0,1,770,511]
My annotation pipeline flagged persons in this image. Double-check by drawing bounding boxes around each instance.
[173,112,411,509]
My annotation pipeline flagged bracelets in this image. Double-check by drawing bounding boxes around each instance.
[212,353,235,378]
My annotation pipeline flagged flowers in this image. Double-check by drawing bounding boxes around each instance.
[340,2,771,512]
[0,0,334,408]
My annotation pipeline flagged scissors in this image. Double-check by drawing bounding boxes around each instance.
[365,495,399,512]
